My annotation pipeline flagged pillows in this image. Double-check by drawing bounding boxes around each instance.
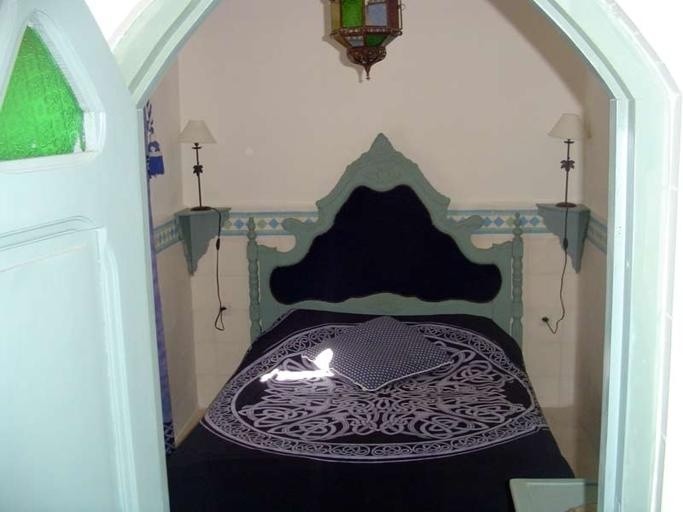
[299,315,455,395]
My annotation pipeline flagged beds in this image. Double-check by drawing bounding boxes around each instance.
[167,133,580,511]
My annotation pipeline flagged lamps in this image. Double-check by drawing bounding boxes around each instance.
[175,121,217,211]
[549,115,589,207]
